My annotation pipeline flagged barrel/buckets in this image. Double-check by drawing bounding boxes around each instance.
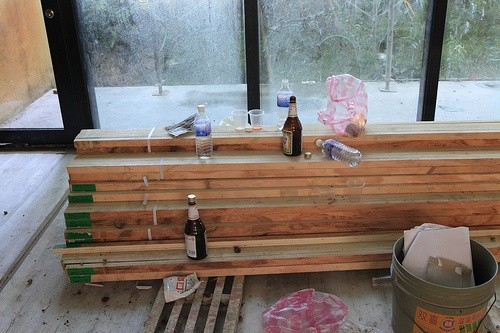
[389,237,498,333]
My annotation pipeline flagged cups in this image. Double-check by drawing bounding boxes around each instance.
[231,110,248,130]
[248,109,264,130]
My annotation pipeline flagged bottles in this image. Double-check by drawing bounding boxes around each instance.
[194,104,213,160]
[282,96,303,157]
[276,78,293,130]
[315,139,361,168]
[185,194,207,260]
